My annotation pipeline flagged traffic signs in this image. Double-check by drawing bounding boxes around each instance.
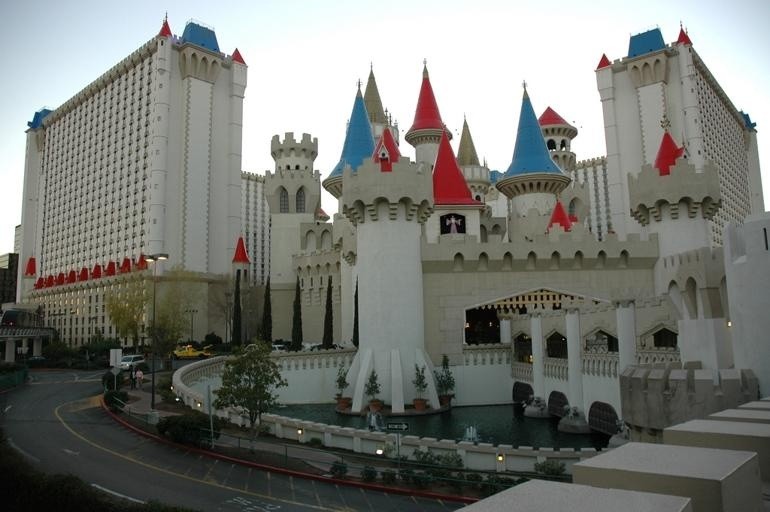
[387,422,410,432]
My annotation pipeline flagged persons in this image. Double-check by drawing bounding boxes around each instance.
[130,368,137,390]
[133,368,146,387]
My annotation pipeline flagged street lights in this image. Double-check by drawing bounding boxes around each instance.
[144,253,169,411]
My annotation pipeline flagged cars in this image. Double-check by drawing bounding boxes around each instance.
[169,344,216,360]
[119,355,145,372]
[271,344,286,352]
